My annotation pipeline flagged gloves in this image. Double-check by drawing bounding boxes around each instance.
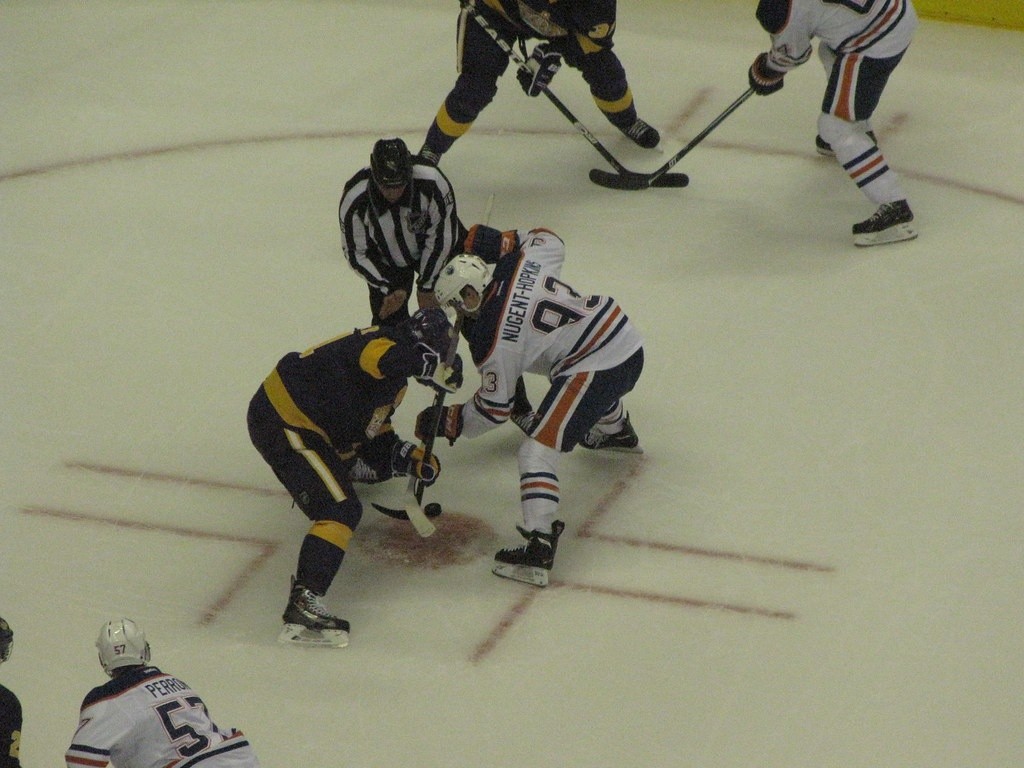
[747,52,784,96]
[396,439,441,486]
[414,402,463,447]
[516,42,562,97]
[412,342,463,394]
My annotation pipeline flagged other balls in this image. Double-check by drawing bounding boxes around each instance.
[424,503,442,517]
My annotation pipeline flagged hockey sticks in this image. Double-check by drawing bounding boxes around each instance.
[459,0,691,188]
[401,358,451,538]
[588,87,759,190]
[371,191,496,519]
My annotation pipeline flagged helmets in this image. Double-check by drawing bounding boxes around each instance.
[433,252,494,330]
[407,306,454,361]
[95,616,151,675]
[0,618,14,663]
[367,137,414,186]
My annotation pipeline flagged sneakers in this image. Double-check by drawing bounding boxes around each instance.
[276,574,351,647]
[509,406,536,435]
[620,115,663,155]
[851,199,918,245]
[815,122,880,158]
[577,408,643,455]
[416,143,442,168]
[490,518,564,587]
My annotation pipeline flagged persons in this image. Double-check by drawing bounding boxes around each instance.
[64,617,260,768]
[0,617,23,768]
[338,138,464,325]
[416,0,664,167]
[414,224,644,588]
[248,306,463,647]
[747,0,918,248]
